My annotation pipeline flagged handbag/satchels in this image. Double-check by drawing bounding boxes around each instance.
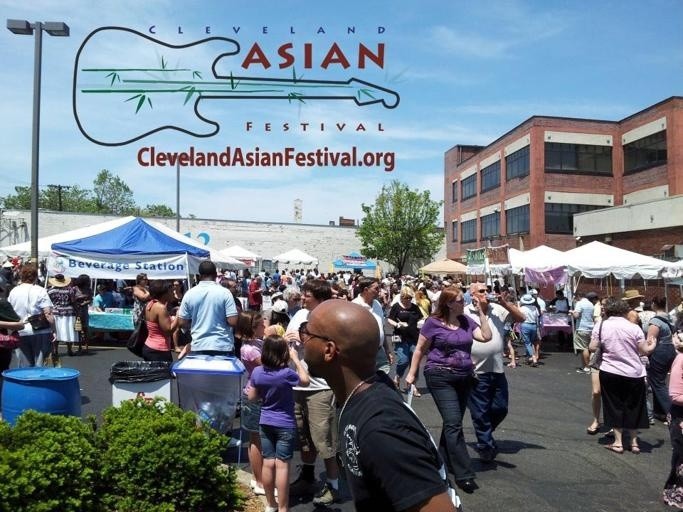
[588,340,603,370]
[28,314,50,330]
[74,318,82,331]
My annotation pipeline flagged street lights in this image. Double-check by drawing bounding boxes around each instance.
[8,18,67,269]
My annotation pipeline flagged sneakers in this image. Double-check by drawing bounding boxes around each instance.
[392,378,401,391]
[289,464,316,495]
[250,478,280,497]
[576,367,590,375]
[528,356,539,367]
[412,387,422,398]
[444,463,479,491]
[264,503,278,512]
[311,483,342,507]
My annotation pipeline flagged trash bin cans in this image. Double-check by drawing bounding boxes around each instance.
[170,354,247,467]
[109,360,176,414]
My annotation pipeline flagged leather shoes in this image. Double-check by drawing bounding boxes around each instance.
[503,352,520,369]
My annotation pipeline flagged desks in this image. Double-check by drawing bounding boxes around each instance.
[88,310,135,332]
[512,318,571,338]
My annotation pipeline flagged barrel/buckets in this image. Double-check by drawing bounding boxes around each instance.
[0,366,82,428]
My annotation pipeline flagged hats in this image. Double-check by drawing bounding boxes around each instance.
[520,293,536,304]
[48,273,72,288]
[272,300,290,314]
[620,289,647,300]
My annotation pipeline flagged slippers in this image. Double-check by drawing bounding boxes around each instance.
[587,422,600,434]
[605,429,625,439]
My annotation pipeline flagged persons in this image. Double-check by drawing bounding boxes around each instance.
[0,261,683,512]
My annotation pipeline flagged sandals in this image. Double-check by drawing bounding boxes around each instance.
[629,443,642,455]
[604,443,625,454]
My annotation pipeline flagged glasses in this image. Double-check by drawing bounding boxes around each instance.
[298,320,329,343]
[475,288,492,294]
